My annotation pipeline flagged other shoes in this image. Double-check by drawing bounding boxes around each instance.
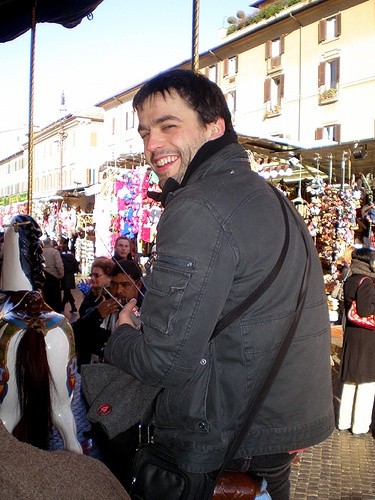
[352,429,372,437]
[69,308,77,313]
[339,428,352,432]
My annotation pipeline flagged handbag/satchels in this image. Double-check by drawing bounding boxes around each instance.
[127,441,217,500]
[346,276,375,331]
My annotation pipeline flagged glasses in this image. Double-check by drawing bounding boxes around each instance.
[89,272,105,278]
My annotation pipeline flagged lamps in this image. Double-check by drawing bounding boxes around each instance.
[353,144,369,161]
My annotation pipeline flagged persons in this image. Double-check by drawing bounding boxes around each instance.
[102,70,335,500]
[38,236,78,314]
[111,236,145,298]
[338,248,375,435]
[78,256,115,317]
[71,260,146,374]
[274,172,375,254]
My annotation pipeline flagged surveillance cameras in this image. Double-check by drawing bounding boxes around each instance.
[353,145,368,160]
